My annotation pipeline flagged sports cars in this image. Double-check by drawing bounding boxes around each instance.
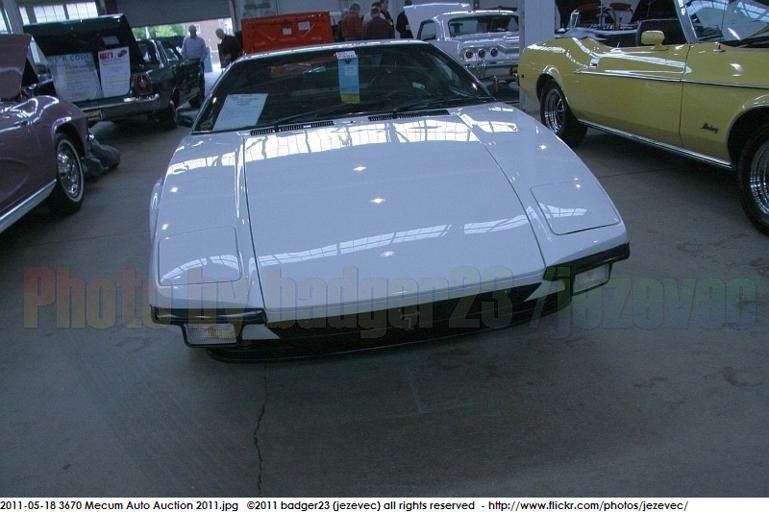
[136,33,641,368]
[515,0,768,240]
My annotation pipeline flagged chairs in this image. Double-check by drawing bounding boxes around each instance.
[632,19,683,47]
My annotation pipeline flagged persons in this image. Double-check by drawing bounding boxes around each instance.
[215,27,243,65]
[179,24,209,109]
[332,0,414,40]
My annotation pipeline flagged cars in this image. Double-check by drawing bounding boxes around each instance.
[1,0,647,123]
[0,28,99,249]
